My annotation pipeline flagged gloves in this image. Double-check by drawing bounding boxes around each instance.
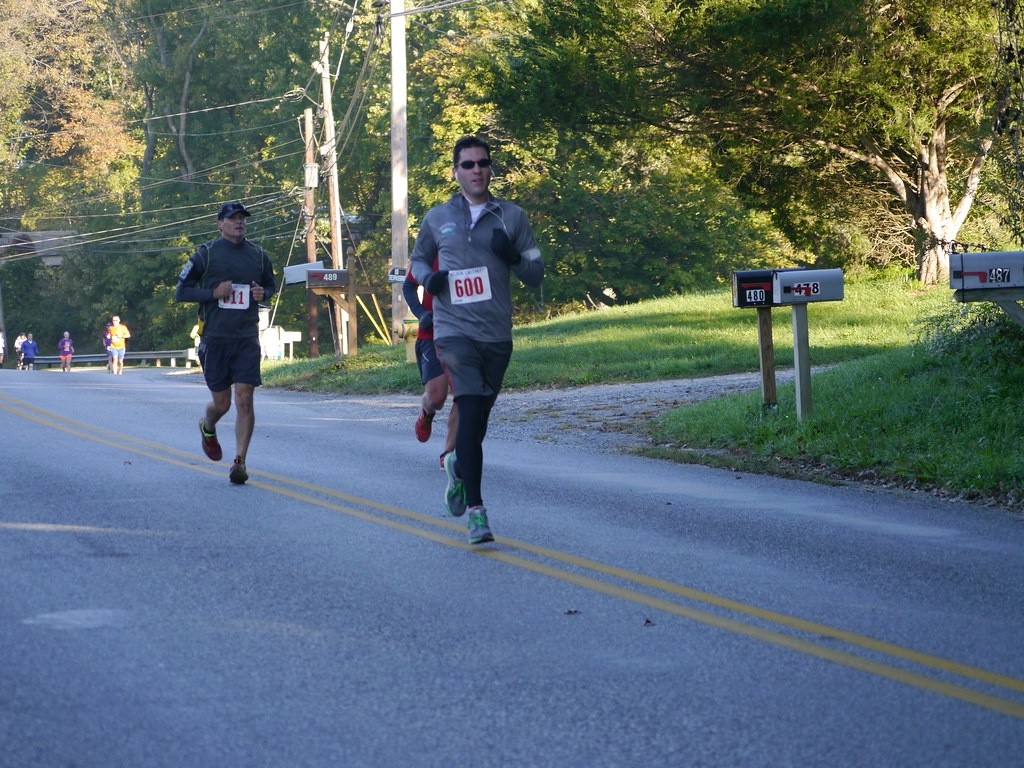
[491,227,520,266]
[424,270,448,297]
[417,310,433,331]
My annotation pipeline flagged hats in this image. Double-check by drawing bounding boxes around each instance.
[217,201,250,220]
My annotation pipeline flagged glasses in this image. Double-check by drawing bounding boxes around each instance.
[452,158,492,170]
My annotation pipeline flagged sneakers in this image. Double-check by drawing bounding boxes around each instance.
[439,449,447,471]
[415,404,435,441]
[443,452,466,517]
[464,506,494,543]
[197,416,222,460]
[228,459,249,484]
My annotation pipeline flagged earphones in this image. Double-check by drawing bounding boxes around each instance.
[455,172,458,179]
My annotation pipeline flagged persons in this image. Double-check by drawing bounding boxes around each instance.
[401,252,463,468]
[176,202,276,484]
[105,315,131,375]
[57,331,74,371]
[14,332,27,366]
[20,332,39,371]
[408,135,544,545]
[102,322,114,373]
[0,331,6,368]
[190,324,203,372]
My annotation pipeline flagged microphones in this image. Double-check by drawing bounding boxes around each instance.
[466,197,472,203]
